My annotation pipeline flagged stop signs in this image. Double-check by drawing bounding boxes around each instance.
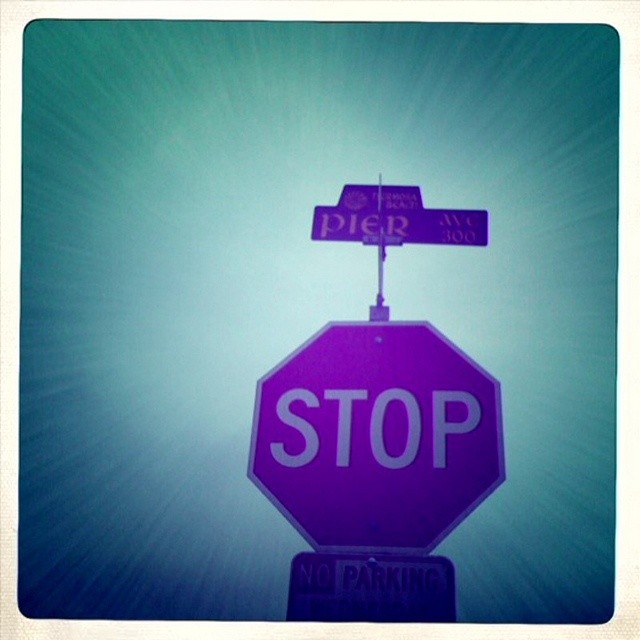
[246,320,507,555]
